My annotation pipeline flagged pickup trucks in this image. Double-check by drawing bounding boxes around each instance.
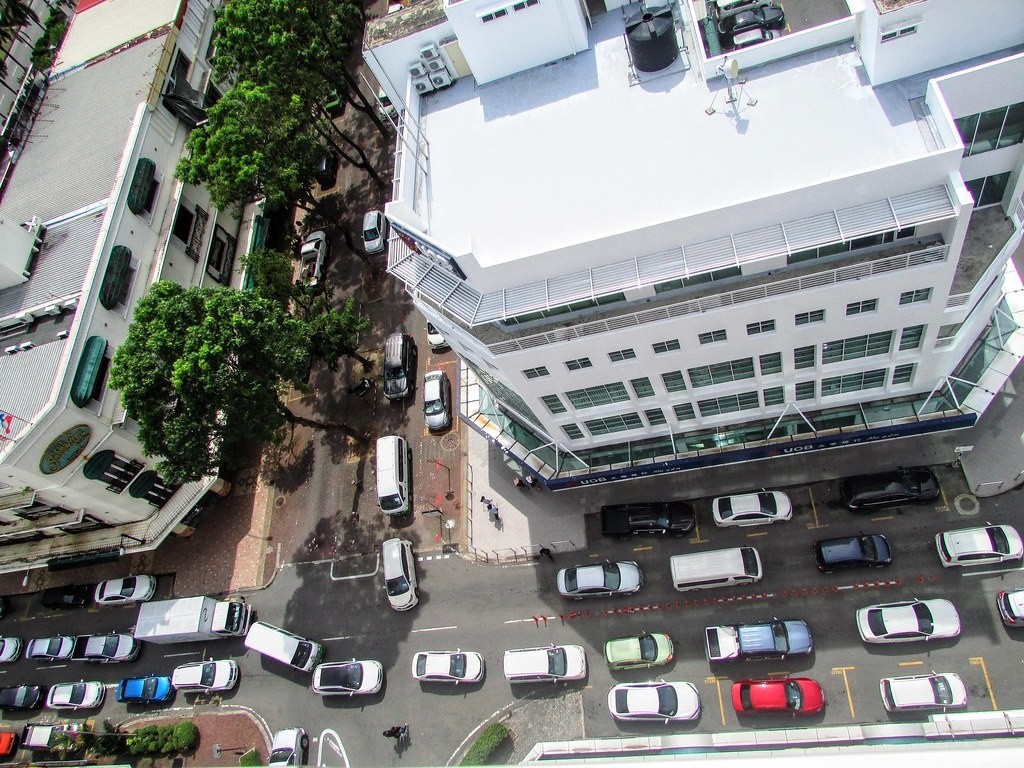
[703,618,811,665]
[21,721,90,753]
[599,502,698,541]
[71,635,142,664]
[296,230,328,288]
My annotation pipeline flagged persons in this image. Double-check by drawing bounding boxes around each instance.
[383,723,408,742]
[513,477,523,489]
[480,496,492,505]
[540,549,553,561]
[486,504,499,521]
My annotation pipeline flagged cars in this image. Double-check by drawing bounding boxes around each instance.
[0,636,22,663]
[713,487,793,530]
[0,683,48,713]
[116,673,176,705]
[93,576,157,608]
[422,369,451,431]
[267,727,309,768]
[386,3,404,13]
[855,594,962,646]
[324,88,346,112]
[556,556,644,602]
[47,679,107,710]
[378,85,397,119]
[731,672,826,717]
[606,677,701,724]
[600,631,674,673]
[25,635,76,662]
[412,646,485,686]
[0,731,17,754]
[996,585,1024,628]
[424,320,450,351]
[42,586,91,610]
[311,658,384,699]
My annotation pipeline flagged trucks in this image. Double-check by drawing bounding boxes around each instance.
[133,596,256,646]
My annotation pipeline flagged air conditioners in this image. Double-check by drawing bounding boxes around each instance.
[15,312,34,325]
[64,298,79,311]
[408,63,426,78]
[426,56,446,73]
[20,341,37,351]
[429,67,453,89]
[3,345,19,355]
[56,330,69,340]
[412,74,434,95]
[44,304,61,316]
[419,44,439,62]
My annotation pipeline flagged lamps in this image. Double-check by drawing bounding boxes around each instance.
[119,533,145,556]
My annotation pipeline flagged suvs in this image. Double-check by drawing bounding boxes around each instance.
[171,657,238,696]
[840,465,942,514]
[360,210,386,254]
[813,531,895,576]
[382,333,416,402]
[501,642,588,686]
[879,667,967,715]
[934,518,1024,571]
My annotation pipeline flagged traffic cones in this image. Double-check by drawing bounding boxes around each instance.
[533,574,944,623]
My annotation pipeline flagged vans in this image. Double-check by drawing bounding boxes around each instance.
[317,149,336,184]
[375,435,411,519]
[381,537,420,612]
[670,545,762,593]
[242,620,324,674]
